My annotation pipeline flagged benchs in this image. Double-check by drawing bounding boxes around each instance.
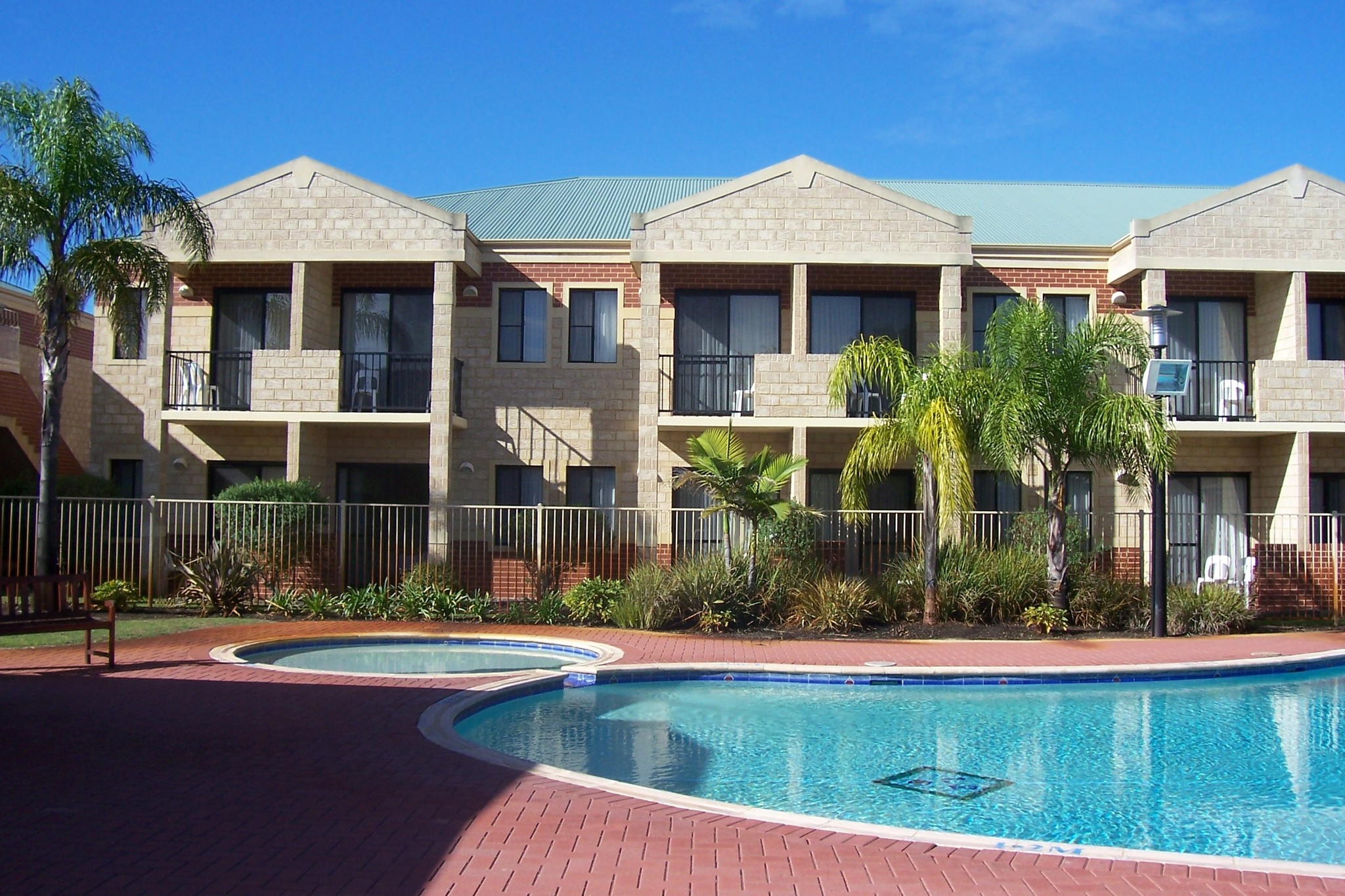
[0,571,117,670]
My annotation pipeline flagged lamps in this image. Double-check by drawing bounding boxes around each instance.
[173,457,188,470]
[462,285,480,297]
[459,461,475,474]
[178,284,195,297]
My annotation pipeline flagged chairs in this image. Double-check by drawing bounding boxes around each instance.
[731,380,756,417]
[176,362,219,412]
[899,370,935,418]
[1196,554,1233,597]
[1218,379,1247,423]
[425,370,459,413]
[847,369,883,419]
[1228,556,1256,609]
[348,368,382,412]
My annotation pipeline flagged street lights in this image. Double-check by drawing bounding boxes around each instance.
[1131,303,1193,638]
